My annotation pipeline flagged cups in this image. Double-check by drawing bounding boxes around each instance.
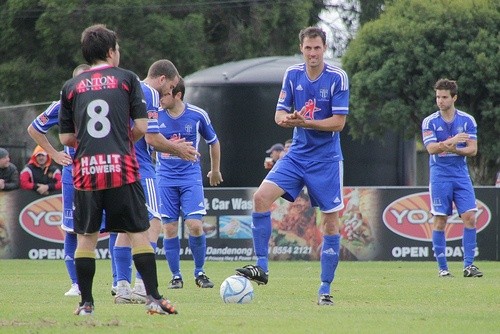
[265,159,272,169]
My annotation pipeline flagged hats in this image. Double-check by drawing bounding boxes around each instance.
[27,144,51,168]
[266,144,284,154]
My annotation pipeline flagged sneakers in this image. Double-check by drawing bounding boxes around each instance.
[114,293,129,304]
[130,291,146,304]
[160,297,178,314]
[463,265,483,277]
[235,264,269,285]
[110,286,118,296]
[439,269,449,277]
[170,277,183,289]
[318,294,334,305]
[74,301,94,316]
[147,302,168,315]
[195,271,214,288]
[64,283,80,296]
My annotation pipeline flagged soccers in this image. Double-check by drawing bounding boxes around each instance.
[219,272,255,304]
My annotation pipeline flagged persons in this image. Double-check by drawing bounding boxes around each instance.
[421,78,483,277]
[236,26,350,306]
[27,24,224,316]
[0,149,20,191]
[264,139,295,168]
[20,145,62,196]
